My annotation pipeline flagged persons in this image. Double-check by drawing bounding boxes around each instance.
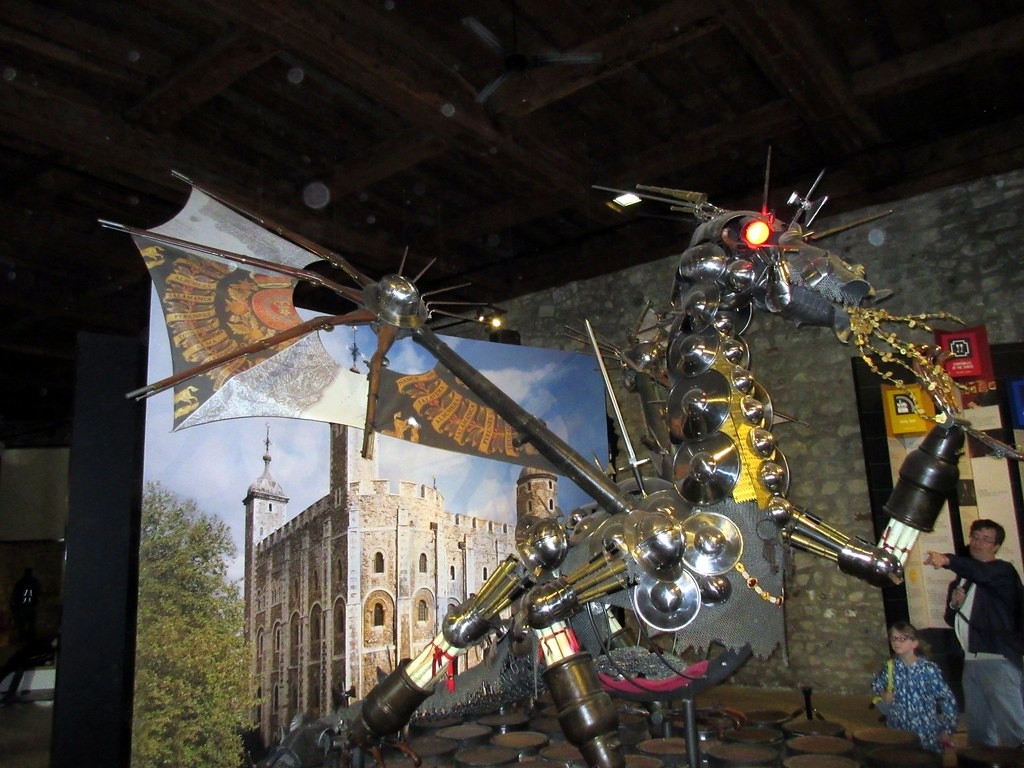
[923,520,1023,768]
[871,621,959,768]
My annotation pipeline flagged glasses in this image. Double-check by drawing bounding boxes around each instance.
[969,535,998,545]
[890,636,913,642]
